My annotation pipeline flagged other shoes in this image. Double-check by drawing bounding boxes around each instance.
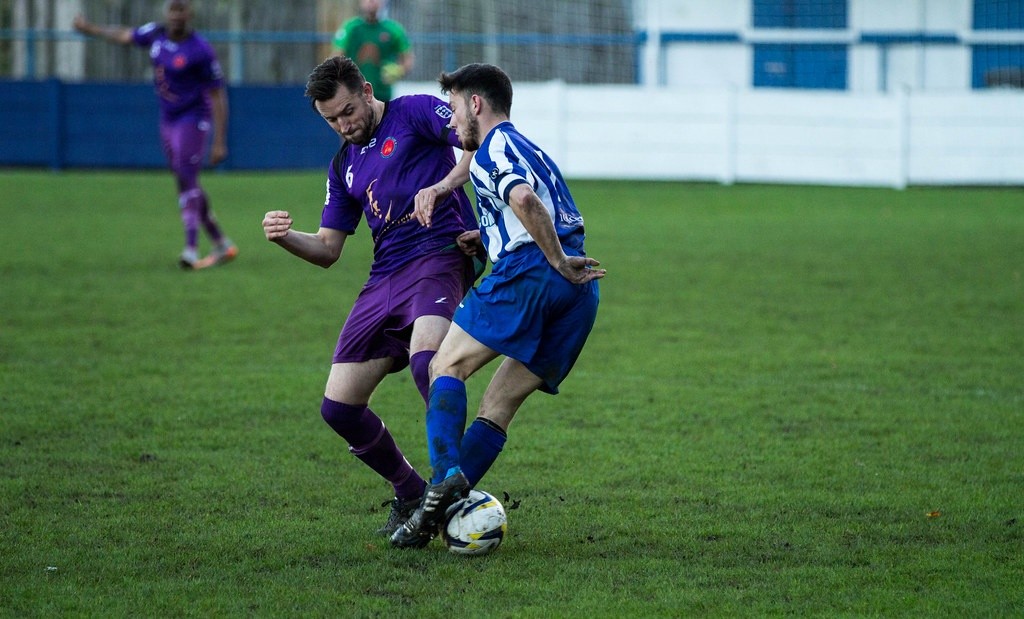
[202,239,237,267]
[178,252,202,270]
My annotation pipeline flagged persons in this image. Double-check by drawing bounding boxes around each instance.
[72,0,239,272]
[261,55,486,536]
[329,0,417,104]
[387,61,608,550]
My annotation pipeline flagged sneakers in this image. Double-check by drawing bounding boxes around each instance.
[389,466,470,548]
[376,480,429,534]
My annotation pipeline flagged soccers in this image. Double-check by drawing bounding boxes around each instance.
[441,488,509,560]
